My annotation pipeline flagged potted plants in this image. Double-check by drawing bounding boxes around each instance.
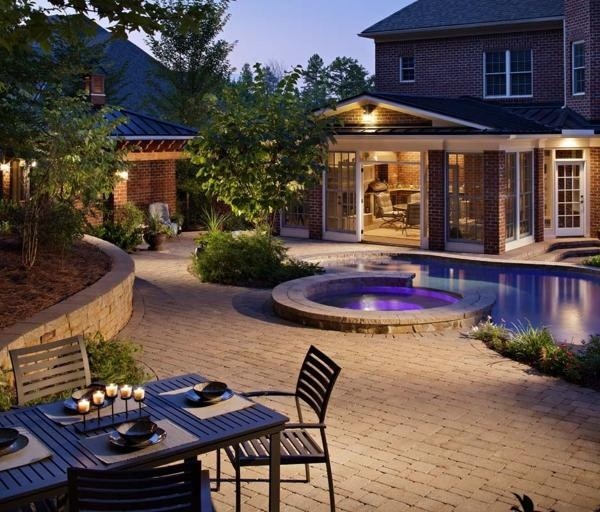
[144,210,166,250]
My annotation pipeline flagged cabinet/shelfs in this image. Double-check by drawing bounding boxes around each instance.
[364,188,420,213]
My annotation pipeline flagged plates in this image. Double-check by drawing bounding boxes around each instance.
[185,389,233,404]
[109,430,167,451]
[0,436,29,455]
[63,395,113,413]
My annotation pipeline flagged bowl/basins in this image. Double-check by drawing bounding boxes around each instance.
[72,389,100,403]
[117,421,156,442]
[193,382,228,399]
[0,428,19,447]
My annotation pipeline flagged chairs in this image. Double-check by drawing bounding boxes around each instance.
[66,461,213,511]
[377,192,420,237]
[9,335,92,406]
[216,344,343,512]
[148,202,178,238]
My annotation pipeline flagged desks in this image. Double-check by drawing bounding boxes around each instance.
[1,371,291,512]
